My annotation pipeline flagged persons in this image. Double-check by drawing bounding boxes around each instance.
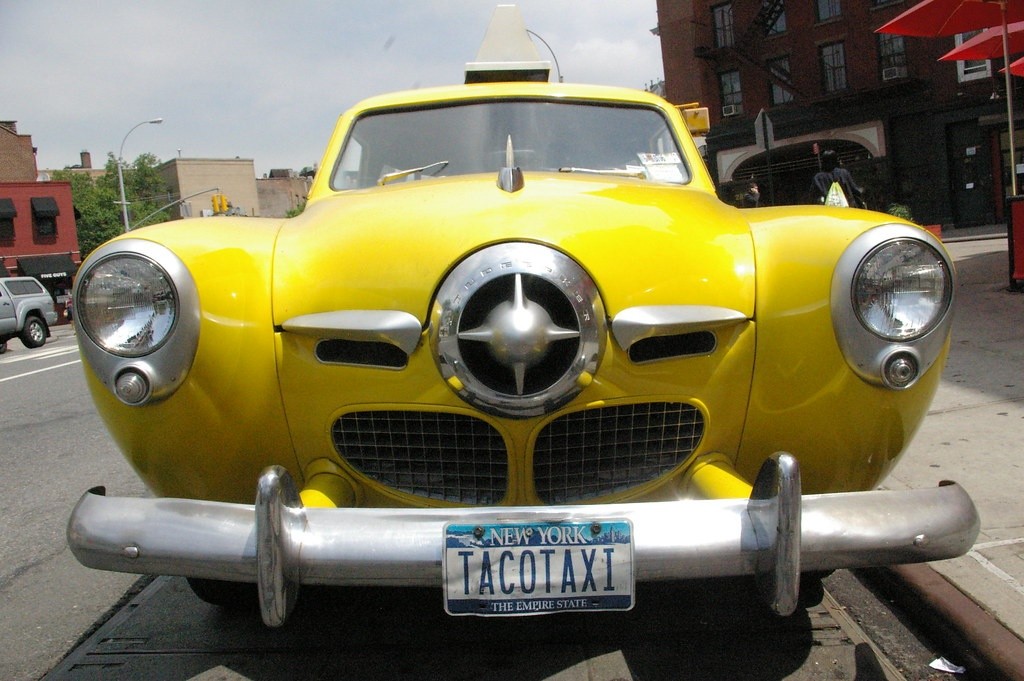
[743,183,760,208]
[65,295,76,329]
[808,150,867,210]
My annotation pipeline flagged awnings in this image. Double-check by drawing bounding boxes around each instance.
[0,255,78,280]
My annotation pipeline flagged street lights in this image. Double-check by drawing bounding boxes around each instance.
[118,118,164,232]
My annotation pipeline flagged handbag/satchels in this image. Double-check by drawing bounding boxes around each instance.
[64,301,68,317]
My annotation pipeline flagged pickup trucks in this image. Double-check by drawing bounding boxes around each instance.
[0,276,59,349]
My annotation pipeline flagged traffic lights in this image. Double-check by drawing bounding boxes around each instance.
[220,193,230,213]
[211,193,224,214]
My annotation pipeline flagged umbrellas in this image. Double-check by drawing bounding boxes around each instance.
[874,0,1024,197]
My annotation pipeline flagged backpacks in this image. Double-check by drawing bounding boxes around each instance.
[824,180,849,208]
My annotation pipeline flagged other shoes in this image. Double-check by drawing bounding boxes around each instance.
[74,333,76,335]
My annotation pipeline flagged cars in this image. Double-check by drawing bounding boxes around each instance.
[69,58,984,617]
